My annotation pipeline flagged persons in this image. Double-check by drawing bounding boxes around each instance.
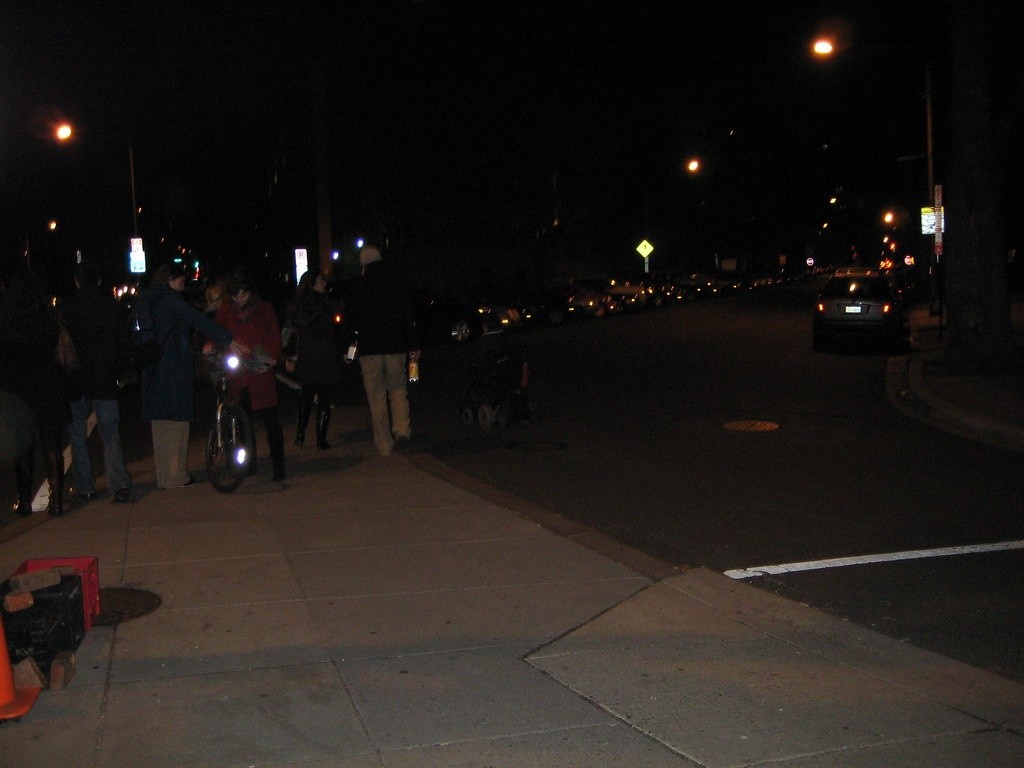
[461,312,528,426]
[1,243,421,515]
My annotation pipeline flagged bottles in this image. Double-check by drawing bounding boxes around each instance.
[409,354,419,383]
[346,330,359,360]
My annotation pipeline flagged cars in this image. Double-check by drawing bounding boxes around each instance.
[884,273,917,312]
[416,274,773,346]
[813,268,903,356]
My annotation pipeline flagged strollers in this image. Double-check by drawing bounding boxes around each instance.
[460,330,541,429]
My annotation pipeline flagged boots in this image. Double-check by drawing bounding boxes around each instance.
[293,407,310,445]
[316,407,333,450]
[15,489,33,516]
[46,490,64,515]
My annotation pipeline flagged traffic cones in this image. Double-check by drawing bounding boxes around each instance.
[0,614,41,721]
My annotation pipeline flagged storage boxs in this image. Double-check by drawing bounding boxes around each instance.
[0,555,100,691]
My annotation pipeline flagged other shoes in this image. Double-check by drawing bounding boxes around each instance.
[110,489,129,502]
[81,493,96,499]
[396,433,410,450]
[179,476,195,488]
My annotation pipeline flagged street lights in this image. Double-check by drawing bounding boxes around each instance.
[57,125,141,284]
[813,41,938,316]
[644,162,697,273]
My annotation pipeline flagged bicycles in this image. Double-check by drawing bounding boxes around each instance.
[205,347,271,493]
